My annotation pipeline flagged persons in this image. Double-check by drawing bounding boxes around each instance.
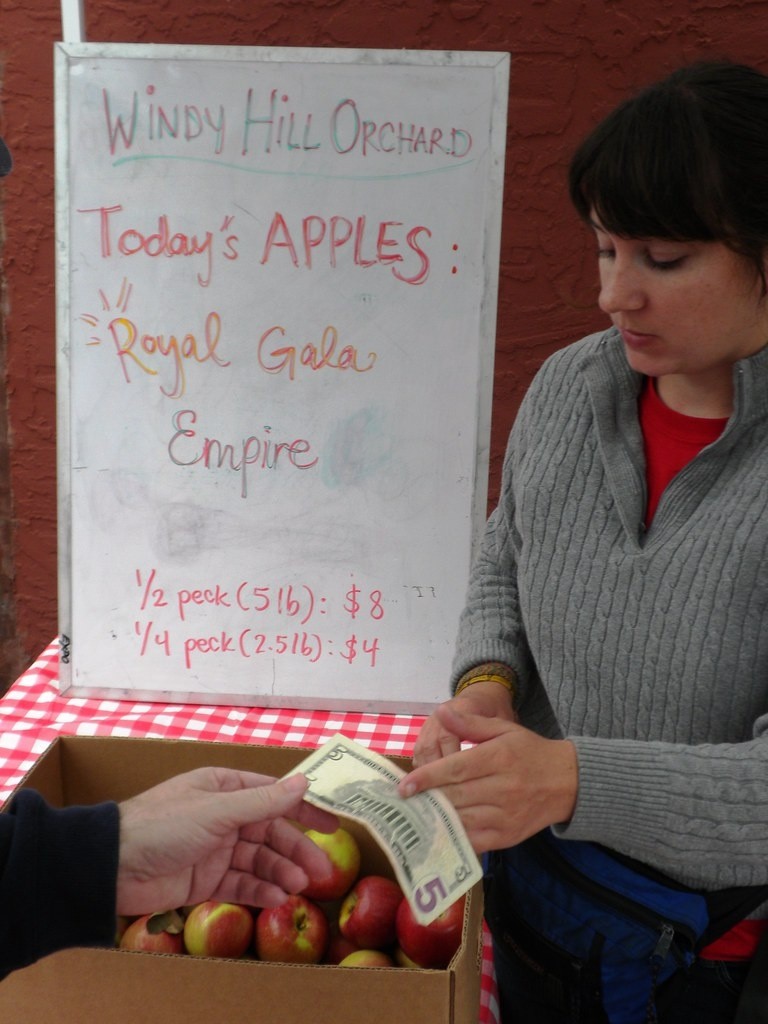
[0,766,341,983]
[402,61,768,1024]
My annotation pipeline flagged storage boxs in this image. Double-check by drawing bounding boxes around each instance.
[0,735,485,1024]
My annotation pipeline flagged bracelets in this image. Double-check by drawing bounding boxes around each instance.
[455,675,514,697]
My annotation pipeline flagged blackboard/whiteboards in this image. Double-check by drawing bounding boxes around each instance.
[47,35,517,721]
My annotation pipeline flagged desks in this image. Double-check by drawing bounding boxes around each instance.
[0,636,502,1024]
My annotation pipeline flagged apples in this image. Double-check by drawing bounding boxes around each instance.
[111,826,467,969]
